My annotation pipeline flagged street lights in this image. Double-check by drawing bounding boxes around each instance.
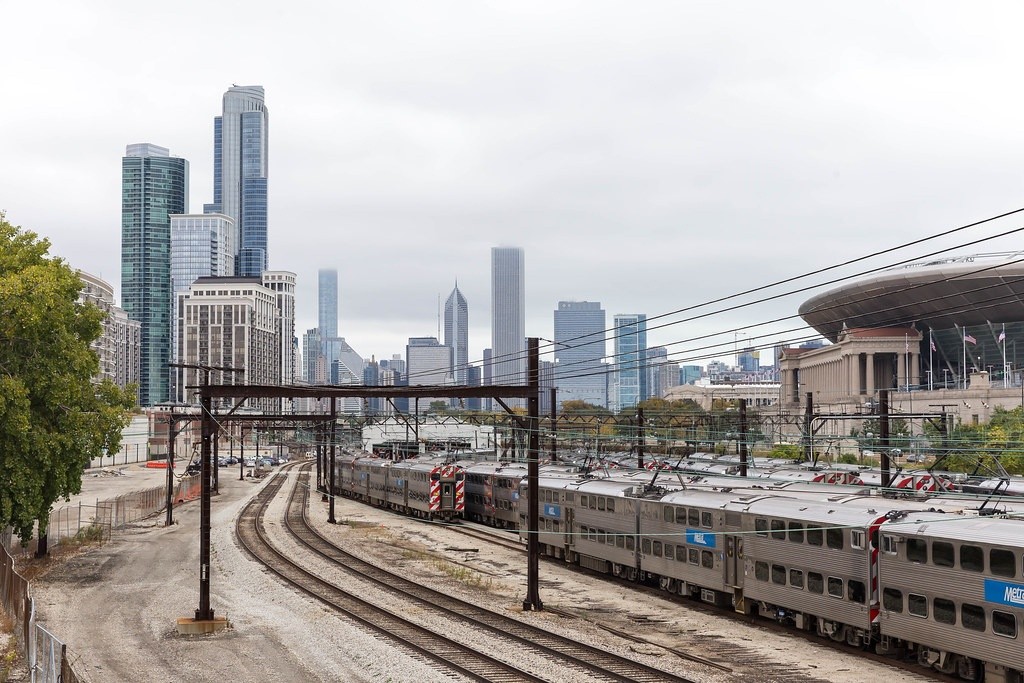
[734,332,746,366]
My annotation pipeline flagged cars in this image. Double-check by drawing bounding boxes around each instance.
[210,455,286,468]
[305,452,314,458]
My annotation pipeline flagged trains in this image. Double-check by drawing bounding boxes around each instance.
[327,450,1023,682]
